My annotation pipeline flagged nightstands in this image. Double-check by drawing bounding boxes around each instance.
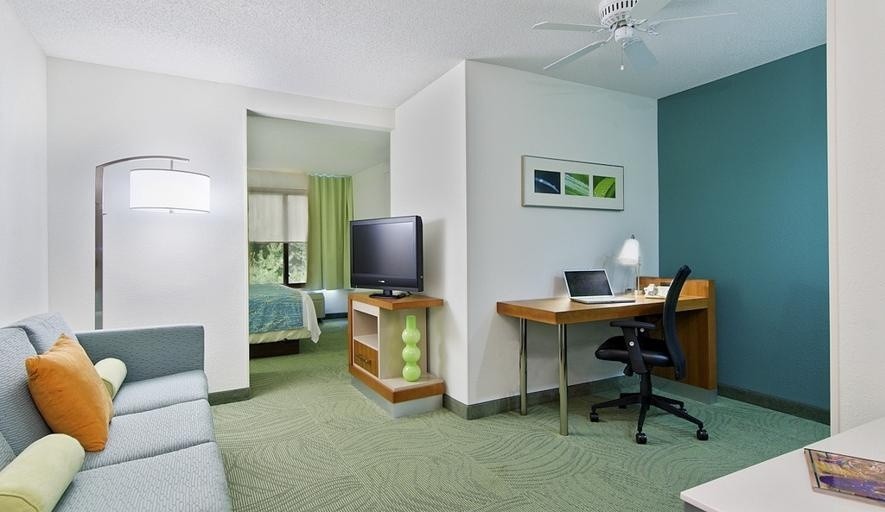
[307,292,325,320]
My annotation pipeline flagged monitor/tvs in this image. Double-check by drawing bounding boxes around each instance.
[349,215,425,300]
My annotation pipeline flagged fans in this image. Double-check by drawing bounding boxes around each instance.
[529,0,739,75]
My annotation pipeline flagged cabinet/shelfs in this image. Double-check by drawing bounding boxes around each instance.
[346,292,447,418]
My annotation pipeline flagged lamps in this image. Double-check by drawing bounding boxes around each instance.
[94,155,212,329]
[615,233,644,298]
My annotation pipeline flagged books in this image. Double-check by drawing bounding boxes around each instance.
[804,447,885,507]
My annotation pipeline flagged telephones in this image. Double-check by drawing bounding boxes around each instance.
[644,283,670,298]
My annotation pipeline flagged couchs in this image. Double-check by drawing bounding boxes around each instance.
[0,312,232,512]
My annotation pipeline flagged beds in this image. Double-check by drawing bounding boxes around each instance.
[249,283,319,360]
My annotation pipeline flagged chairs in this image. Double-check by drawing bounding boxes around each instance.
[589,265,708,444]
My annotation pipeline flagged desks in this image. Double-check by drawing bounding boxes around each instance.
[496,276,718,436]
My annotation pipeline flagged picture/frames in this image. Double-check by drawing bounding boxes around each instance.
[520,154,625,211]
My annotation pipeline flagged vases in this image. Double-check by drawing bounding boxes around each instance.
[401,315,421,383]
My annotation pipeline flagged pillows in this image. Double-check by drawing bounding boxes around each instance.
[27,331,114,453]
[0,432,86,512]
[93,356,127,401]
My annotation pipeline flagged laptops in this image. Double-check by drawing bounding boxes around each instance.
[563,270,637,303]
